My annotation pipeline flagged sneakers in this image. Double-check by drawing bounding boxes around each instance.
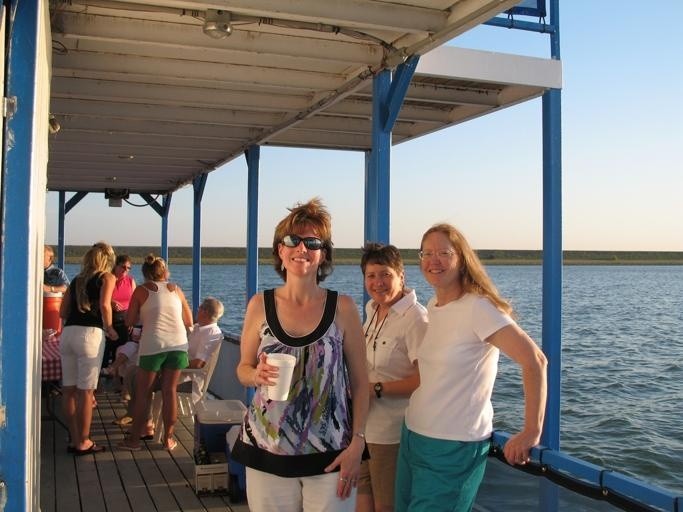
[99,366,118,379]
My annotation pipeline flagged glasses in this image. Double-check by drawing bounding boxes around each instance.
[278,234,328,249]
[118,263,131,271]
[418,249,456,259]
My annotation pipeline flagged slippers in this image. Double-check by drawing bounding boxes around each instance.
[162,439,178,451]
[121,427,156,440]
[115,383,129,394]
[115,439,143,452]
[67,440,107,457]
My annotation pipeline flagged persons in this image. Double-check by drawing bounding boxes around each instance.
[41,246,71,397]
[360,242,428,510]
[392,222,550,510]
[112,296,225,440]
[59,242,119,455]
[229,196,370,512]
[100,343,139,404]
[117,251,193,451]
[101,254,137,368]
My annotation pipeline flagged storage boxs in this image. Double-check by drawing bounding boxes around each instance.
[192,396,247,501]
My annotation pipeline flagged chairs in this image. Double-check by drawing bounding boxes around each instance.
[151,337,223,443]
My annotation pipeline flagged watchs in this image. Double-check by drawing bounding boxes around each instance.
[372,382,385,403]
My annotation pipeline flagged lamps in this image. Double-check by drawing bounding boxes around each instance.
[200,7,234,42]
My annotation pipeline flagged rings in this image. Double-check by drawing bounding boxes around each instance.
[350,477,357,484]
[339,477,348,485]
[513,455,521,460]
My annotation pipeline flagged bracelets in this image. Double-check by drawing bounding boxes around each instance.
[352,430,365,442]
[51,286,54,293]
[252,368,262,390]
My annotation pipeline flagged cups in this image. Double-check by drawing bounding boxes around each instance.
[263,351,296,404]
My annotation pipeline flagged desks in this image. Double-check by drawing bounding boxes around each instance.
[40,333,72,441]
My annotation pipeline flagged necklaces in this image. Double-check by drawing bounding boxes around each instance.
[364,302,388,371]
